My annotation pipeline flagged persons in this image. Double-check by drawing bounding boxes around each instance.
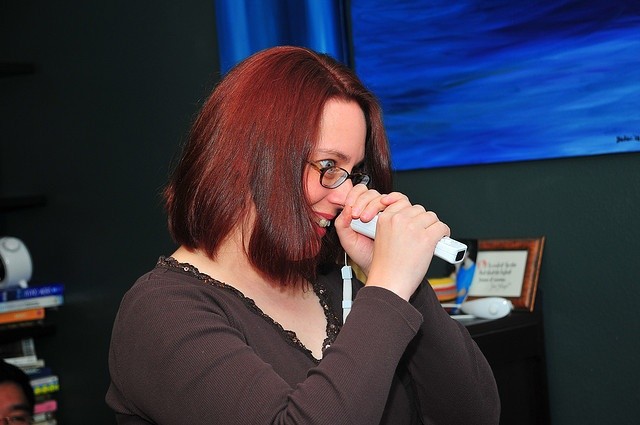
[99,46,504,423]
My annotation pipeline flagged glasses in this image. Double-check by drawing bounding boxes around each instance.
[275,144,371,199]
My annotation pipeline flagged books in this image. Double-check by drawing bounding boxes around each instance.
[0,284,64,302]
[28,366,61,425]
[0,309,45,324]
[0,295,62,314]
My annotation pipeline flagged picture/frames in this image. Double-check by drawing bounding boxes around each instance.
[464,237,548,312]
